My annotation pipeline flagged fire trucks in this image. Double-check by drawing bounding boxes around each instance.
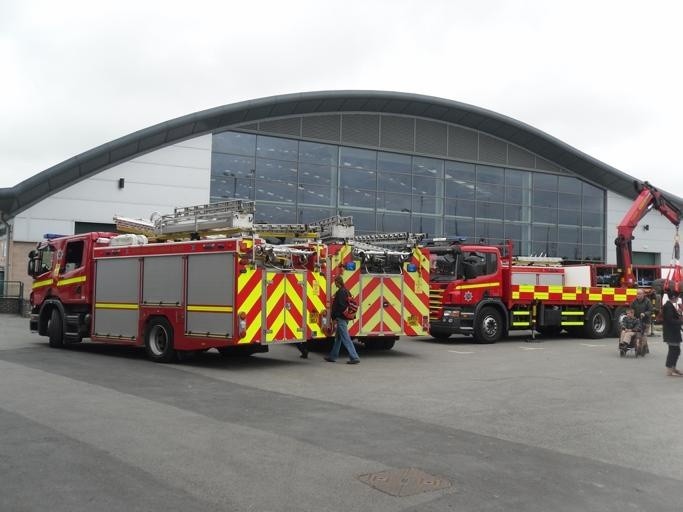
[291,216,430,352]
[26,199,332,362]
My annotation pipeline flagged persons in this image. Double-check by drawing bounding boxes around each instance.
[323,274,360,364]
[296,342,310,358]
[619,288,683,376]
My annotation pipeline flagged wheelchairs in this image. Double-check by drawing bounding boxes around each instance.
[615,315,647,357]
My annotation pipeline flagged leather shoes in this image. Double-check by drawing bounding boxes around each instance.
[324,357,336,362]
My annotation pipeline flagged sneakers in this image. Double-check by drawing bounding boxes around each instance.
[671,370,683,376]
[347,361,360,364]
[667,368,680,375]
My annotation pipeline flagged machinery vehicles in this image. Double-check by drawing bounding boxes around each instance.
[421,182,683,342]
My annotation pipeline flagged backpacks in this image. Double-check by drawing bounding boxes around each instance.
[337,289,359,321]
